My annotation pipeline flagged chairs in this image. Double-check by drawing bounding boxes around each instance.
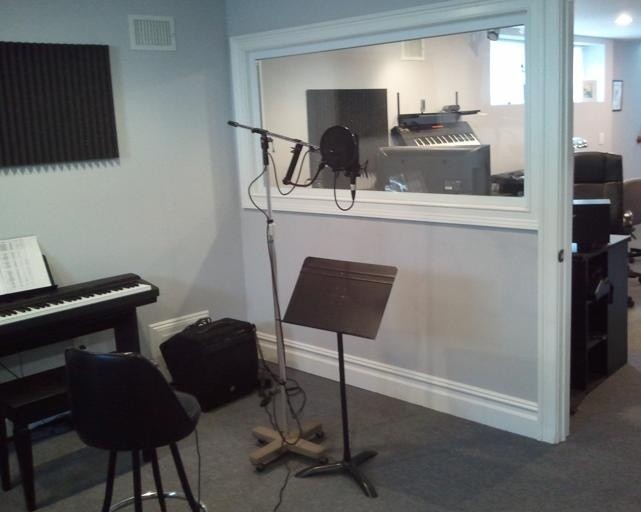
[65,347,209,512]
[574,152,641,307]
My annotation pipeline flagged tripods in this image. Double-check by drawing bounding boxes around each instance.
[294,334,378,498]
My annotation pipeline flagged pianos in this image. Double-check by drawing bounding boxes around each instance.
[390,121,481,147]
[0,272,159,339]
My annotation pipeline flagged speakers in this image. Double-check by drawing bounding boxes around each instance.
[159,317,259,413]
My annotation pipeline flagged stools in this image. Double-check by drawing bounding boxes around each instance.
[0,365,156,512]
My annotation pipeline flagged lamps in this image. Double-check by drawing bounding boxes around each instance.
[488,26,501,41]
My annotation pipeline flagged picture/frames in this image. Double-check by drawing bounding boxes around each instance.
[613,80,623,112]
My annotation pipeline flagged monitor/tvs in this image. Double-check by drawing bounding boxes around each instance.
[376,142,492,195]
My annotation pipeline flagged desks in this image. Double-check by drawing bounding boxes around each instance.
[572,234,631,412]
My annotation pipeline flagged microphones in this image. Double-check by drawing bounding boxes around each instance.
[350,133,359,200]
